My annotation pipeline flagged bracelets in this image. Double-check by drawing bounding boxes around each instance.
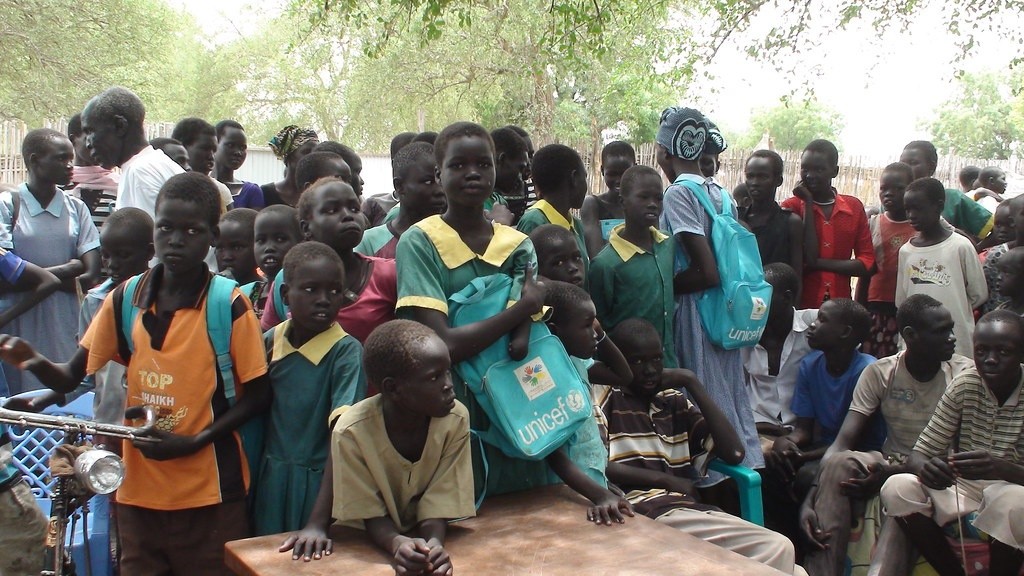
[598,331,607,342]
[810,483,818,488]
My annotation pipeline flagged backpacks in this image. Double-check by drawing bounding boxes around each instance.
[446,272,592,461]
[663,180,773,350]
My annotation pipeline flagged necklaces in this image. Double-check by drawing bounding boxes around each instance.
[827,351,852,372]
[907,348,939,381]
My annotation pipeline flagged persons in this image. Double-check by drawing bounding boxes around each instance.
[512,106,1024,470]
[740,260,862,547]
[796,293,978,574]
[1,170,275,576]
[877,306,1024,576]
[591,314,810,576]
[485,278,636,526]
[330,318,479,575]
[241,241,369,562]
[0,83,552,452]
[771,297,889,565]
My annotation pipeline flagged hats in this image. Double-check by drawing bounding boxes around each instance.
[656,106,709,160]
[703,117,728,154]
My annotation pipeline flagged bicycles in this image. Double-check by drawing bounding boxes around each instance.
[1,390,162,575]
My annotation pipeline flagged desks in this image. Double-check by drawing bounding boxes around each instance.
[224,482,793,576]
